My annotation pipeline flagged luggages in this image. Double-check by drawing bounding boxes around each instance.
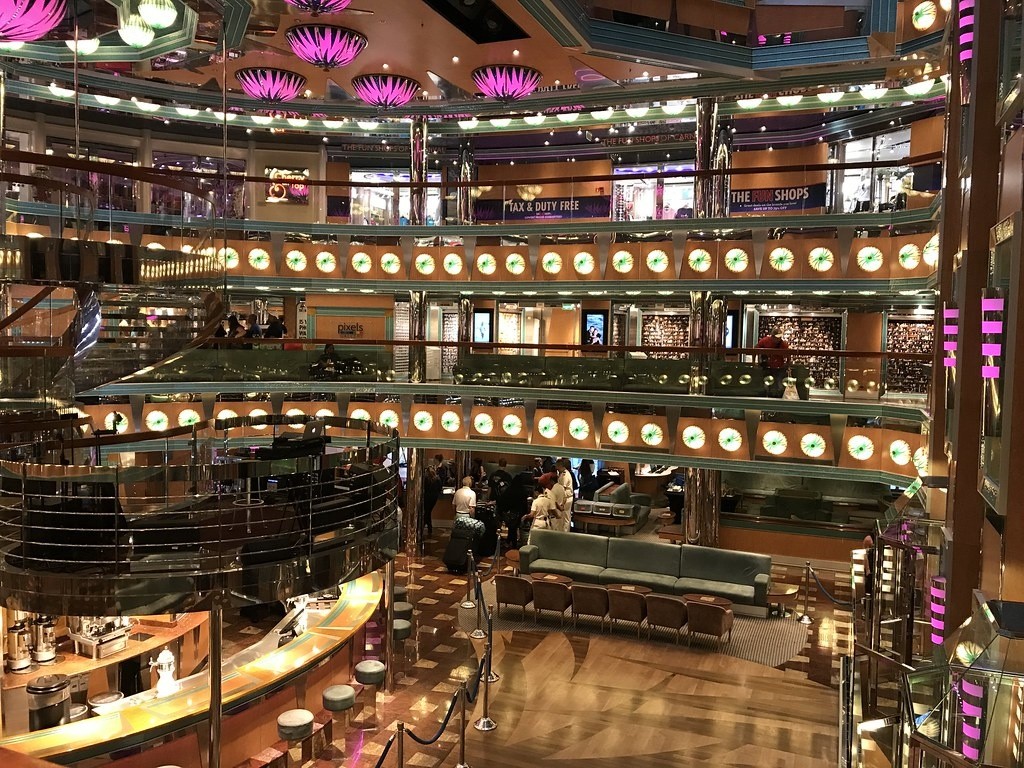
[444,519,485,574]
[476,507,496,555]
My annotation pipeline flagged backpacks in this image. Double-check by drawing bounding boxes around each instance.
[495,479,510,495]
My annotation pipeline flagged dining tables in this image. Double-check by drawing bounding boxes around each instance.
[607,581,654,615]
[529,570,574,603]
[681,593,737,624]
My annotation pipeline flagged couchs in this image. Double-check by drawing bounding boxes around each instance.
[519,527,771,619]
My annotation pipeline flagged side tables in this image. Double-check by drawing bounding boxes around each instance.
[767,579,800,620]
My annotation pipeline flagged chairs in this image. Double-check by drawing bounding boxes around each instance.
[494,572,736,652]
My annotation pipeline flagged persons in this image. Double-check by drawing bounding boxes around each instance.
[216,313,287,350]
[321,344,339,363]
[752,326,790,398]
[424,452,595,545]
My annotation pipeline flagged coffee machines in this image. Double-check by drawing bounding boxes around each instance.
[31,612,58,662]
[6,615,31,670]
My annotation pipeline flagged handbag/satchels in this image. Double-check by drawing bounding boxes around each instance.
[453,517,485,541]
